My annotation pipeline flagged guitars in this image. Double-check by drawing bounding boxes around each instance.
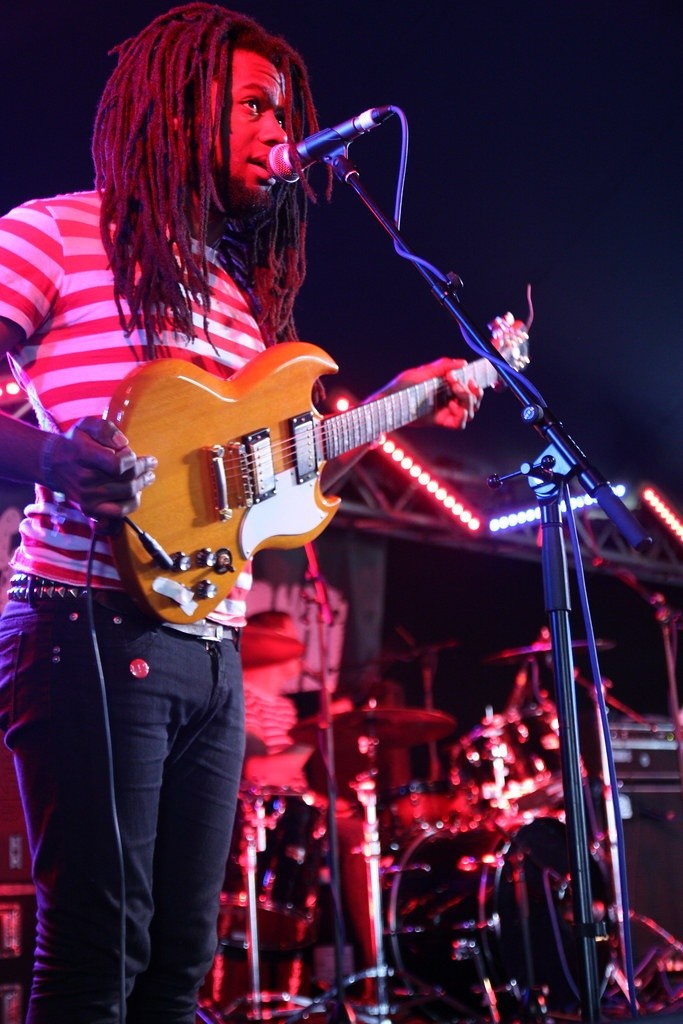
[105,310,531,624]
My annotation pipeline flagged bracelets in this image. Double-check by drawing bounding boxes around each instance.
[41,435,66,492]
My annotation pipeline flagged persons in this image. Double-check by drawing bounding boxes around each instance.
[1,0,485,1024]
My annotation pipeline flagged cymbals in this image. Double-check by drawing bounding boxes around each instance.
[483,626,613,673]
[286,704,456,747]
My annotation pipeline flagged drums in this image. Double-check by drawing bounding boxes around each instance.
[386,816,620,1024]
[217,783,328,954]
[446,698,589,823]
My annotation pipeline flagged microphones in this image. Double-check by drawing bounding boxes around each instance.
[266,104,393,187]
